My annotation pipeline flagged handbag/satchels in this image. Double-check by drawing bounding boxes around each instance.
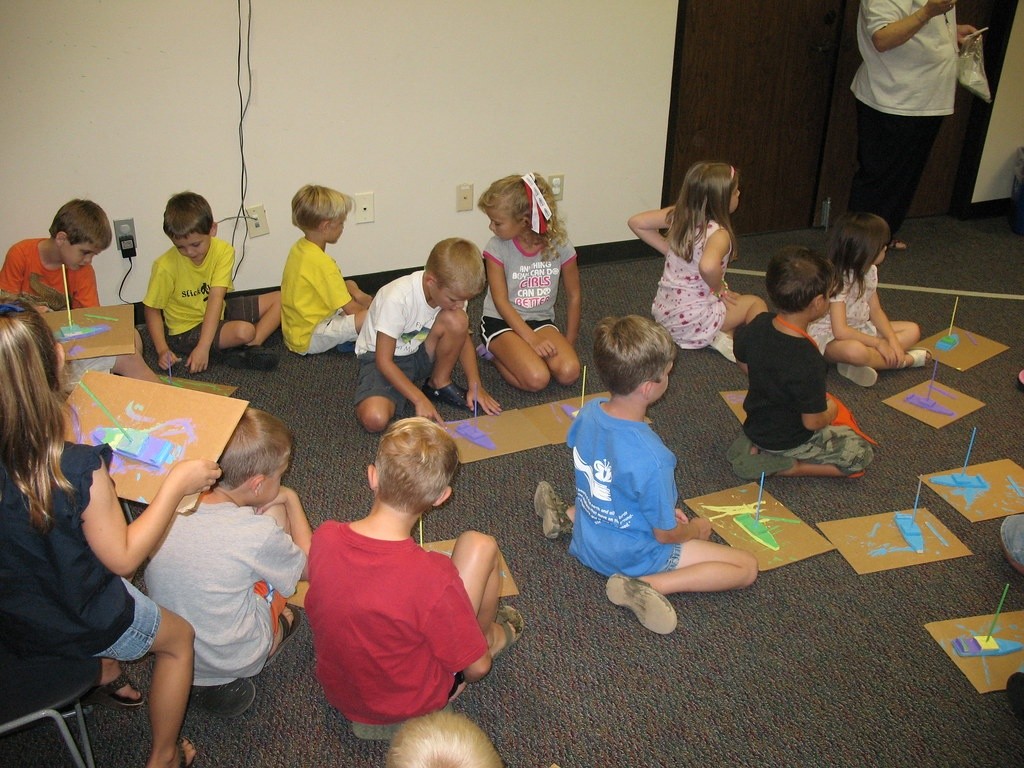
[957,31,992,104]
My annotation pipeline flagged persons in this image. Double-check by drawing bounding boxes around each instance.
[534,314,759,635]
[305,415,523,741]
[802,213,932,387]
[142,191,282,373]
[0,199,164,391]
[350,237,502,434]
[475,173,581,393]
[842,0,981,248]
[144,409,313,720]
[0,295,224,768]
[386,712,505,768]
[728,246,874,478]
[285,185,374,356]
[1000,514,1024,576]
[628,162,770,364]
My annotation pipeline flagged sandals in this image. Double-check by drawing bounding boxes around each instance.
[837,362,877,388]
[905,348,932,367]
[423,377,468,408]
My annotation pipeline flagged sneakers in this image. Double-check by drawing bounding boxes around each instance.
[606,574,677,634]
[534,481,573,539]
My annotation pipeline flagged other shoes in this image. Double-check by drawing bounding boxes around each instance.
[710,330,737,364]
[476,343,494,359]
[337,341,356,352]
[225,345,280,370]
[733,452,796,479]
[727,431,751,463]
[888,239,907,250]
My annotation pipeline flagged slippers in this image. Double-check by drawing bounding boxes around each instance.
[175,736,197,768]
[484,604,523,660]
[352,702,455,740]
[80,674,144,710]
[262,604,303,666]
[203,678,256,719]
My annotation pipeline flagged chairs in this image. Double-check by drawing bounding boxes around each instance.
[0,622,106,768]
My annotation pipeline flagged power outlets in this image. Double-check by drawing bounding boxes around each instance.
[454,182,474,212]
[548,173,564,200]
[246,202,271,239]
[354,190,375,225]
[113,217,137,251]
